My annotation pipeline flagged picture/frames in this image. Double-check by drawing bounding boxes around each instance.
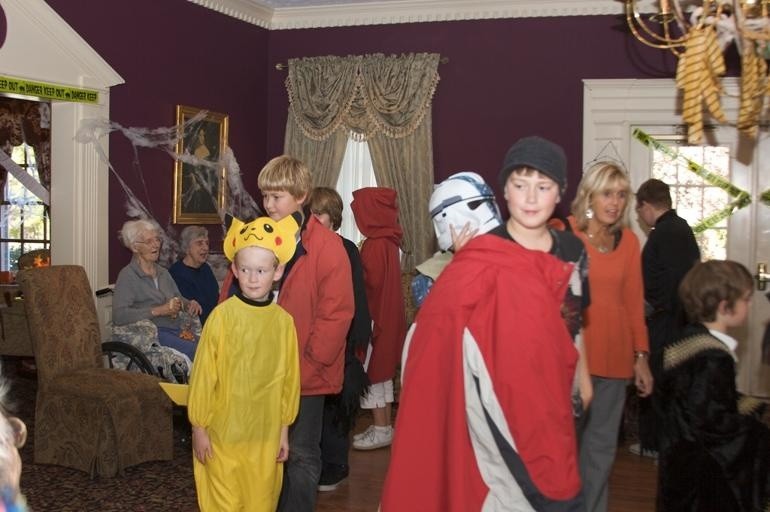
[173,104,230,224]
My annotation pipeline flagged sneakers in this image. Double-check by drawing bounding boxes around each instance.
[629,442,661,461]
[354,425,395,451]
[317,463,350,491]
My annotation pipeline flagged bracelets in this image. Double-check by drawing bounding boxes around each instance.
[635,353,651,358]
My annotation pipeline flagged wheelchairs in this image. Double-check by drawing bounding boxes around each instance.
[95,283,210,452]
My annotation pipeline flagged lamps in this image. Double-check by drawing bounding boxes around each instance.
[613,0,770,146]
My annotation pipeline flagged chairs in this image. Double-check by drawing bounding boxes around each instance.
[19,249,50,269]
[18,264,175,477]
[1,297,33,356]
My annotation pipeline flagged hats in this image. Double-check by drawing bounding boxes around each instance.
[224,216,299,267]
[498,136,567,190]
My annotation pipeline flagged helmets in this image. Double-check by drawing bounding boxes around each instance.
[428,172,501,252]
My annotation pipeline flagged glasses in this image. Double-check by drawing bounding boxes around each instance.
[132,237,164,246]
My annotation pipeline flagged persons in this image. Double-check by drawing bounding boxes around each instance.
[626,178,700,460]
[376,136,594,512]
[656,259,770,512]
[158,211,302,512]
[350,186,408,451]
[410,170,504,317]
[309,186,372,492]
[168,225,220,329]
[112,220,203,363]
[546,159,654,512]
[185,126,217,212]
[217,155,356,512]
